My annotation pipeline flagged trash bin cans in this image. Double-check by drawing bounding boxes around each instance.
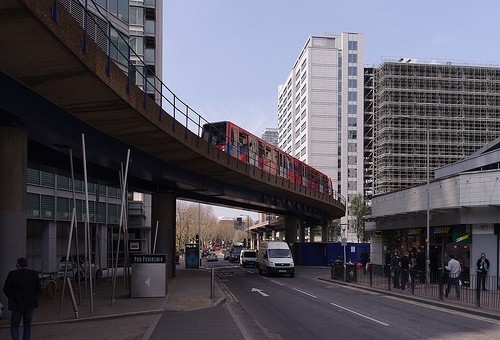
[331,260,344,280]
[346,263,357,282]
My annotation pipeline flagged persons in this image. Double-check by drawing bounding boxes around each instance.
[176,249,184,262]
[4,257,40,340]
[361,247,489,301]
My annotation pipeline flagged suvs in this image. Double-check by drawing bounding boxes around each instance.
[59,254,102,281]
[239,250,259,268]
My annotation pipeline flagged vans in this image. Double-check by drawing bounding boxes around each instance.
[259,241,295,278]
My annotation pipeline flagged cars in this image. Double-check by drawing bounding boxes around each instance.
[201,244,242,263]
[207,253,218,261]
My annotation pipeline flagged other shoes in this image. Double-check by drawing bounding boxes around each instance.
[456,297,460,300]
[482,288,487,291]
[445,295,448,298]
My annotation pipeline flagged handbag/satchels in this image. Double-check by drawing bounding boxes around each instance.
[459,279,462,286]
[484,263,488,271]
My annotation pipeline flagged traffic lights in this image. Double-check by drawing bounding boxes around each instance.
[195,235,200,243]
